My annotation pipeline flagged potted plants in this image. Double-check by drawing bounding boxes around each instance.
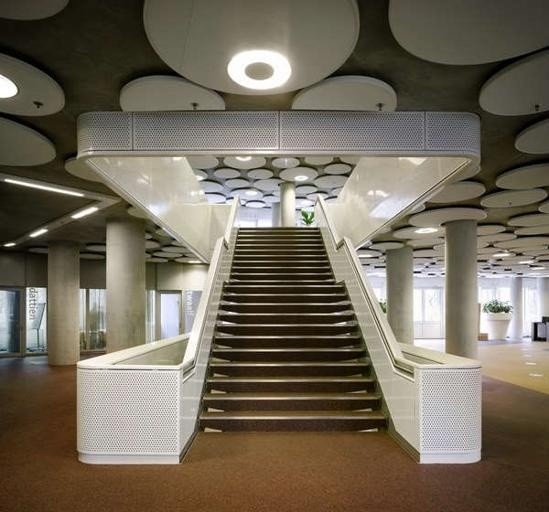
[481,299,514,341]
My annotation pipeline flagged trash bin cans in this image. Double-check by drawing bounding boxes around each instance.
[532,322,546,341]
